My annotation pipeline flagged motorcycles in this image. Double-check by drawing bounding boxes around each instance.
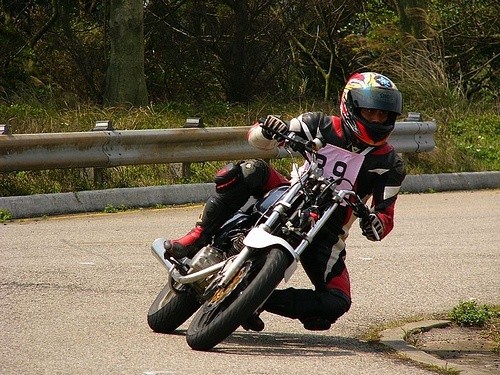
[147,117,380,351]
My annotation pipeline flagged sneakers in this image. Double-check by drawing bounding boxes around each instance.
[248,314,265,332]
[164,227,206,258]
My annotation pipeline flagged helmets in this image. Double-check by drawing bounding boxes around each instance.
[340,72,402,148]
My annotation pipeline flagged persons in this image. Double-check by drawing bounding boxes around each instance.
[164,72,407,330]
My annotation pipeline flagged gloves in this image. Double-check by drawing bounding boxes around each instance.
[359,213,384,241]
[262,114,288,140]
